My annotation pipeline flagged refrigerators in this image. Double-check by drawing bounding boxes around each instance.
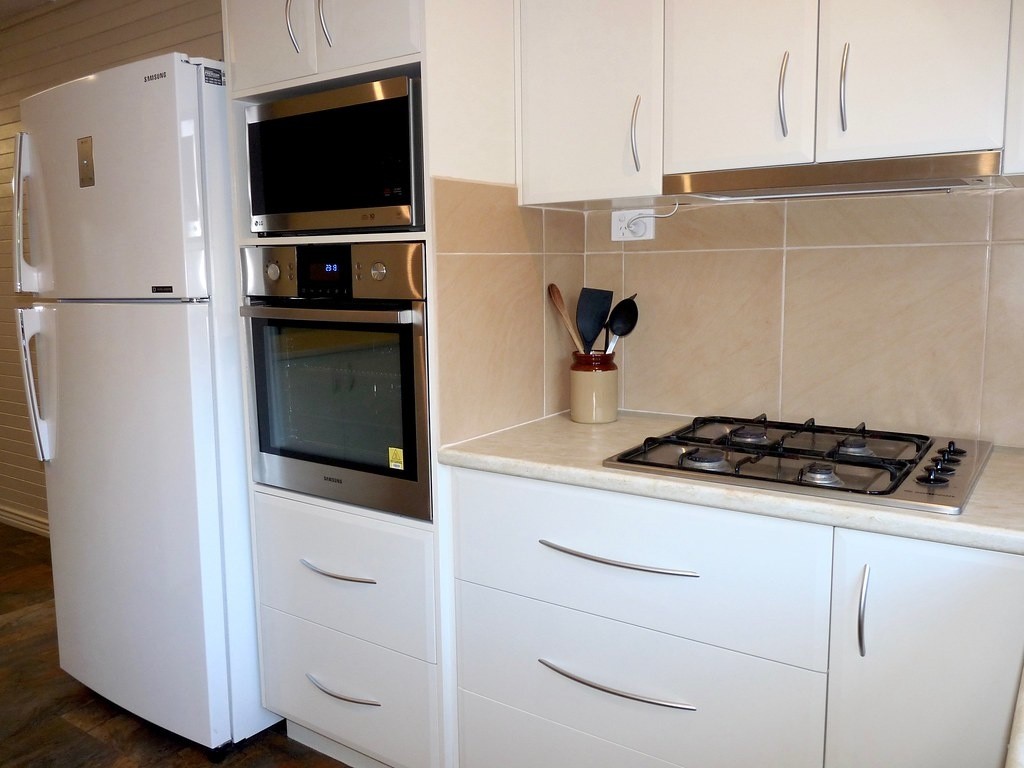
[11,52,284,749]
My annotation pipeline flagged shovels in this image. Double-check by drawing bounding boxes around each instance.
[576,285,615,355]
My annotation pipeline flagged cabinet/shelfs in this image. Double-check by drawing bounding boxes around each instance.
[222,0,421,103]
[664,0,1012,205]
[822,527,1023,768]
[512,1,665,212]
[450,467,834,768]
[249,485,459,768]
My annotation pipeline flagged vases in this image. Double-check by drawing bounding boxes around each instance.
[568,351,618,423]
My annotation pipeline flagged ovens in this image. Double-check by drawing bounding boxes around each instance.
[239,242,433,523]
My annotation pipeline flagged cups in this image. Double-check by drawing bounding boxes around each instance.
[568,351,618,423]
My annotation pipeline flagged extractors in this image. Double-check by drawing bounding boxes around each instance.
[662,150,1013,202]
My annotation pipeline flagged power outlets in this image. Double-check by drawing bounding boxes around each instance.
[611,209,655,241]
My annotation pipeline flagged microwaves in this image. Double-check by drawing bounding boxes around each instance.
[245,73,425,238]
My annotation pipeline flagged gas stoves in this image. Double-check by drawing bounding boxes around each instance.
[602,416,993,515]
